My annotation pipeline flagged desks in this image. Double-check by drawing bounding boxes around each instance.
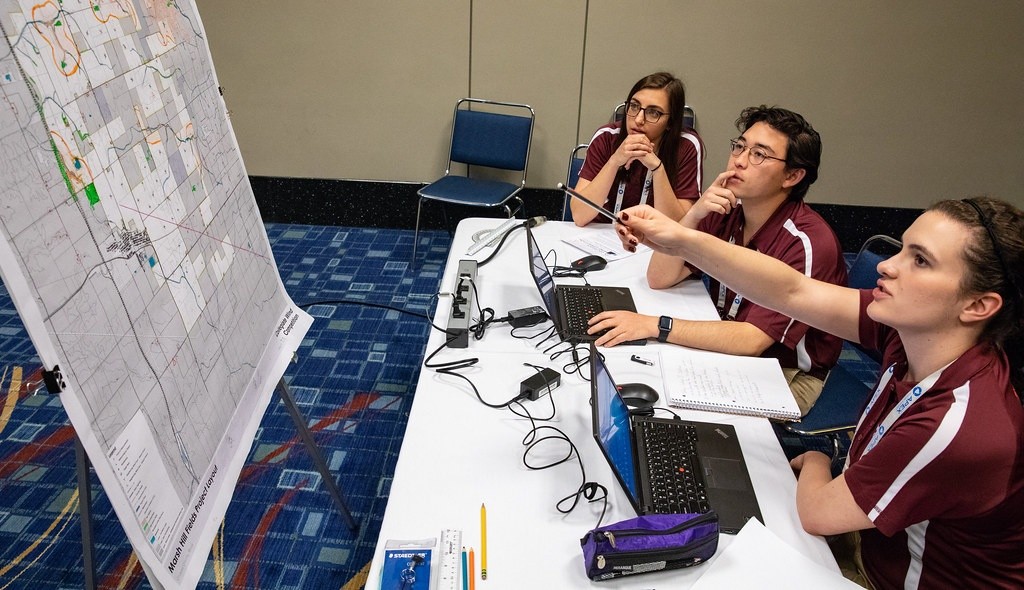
[366,217,845,590]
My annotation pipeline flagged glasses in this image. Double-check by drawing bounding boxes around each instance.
[729,138,790,165]
[624,101,672,124]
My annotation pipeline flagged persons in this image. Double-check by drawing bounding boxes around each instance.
[613,197,1024,590]
[571,72,704,227]
[587,105,848,418]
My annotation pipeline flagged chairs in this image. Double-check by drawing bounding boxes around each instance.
[771,236,906,475]
[413,97,535,264]
[561,103,697,220]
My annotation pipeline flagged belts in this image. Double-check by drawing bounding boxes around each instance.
[805,366,829,381]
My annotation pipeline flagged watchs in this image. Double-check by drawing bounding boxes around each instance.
[657,316,673,343]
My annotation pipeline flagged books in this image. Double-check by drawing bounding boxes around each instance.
[658,345,801,423]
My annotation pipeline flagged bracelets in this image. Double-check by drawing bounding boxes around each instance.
[651,161,661,171]
